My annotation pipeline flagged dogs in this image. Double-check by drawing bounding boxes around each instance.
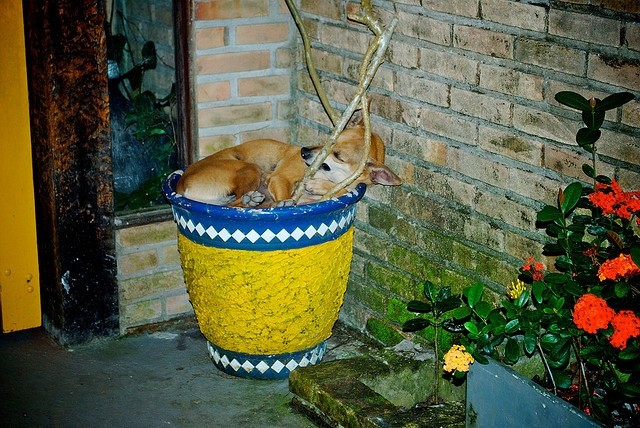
[175,94,403,209]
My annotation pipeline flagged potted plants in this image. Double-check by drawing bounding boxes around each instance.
[272,380,274,383]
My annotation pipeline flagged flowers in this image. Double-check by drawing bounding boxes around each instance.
[403,89,640,428]
